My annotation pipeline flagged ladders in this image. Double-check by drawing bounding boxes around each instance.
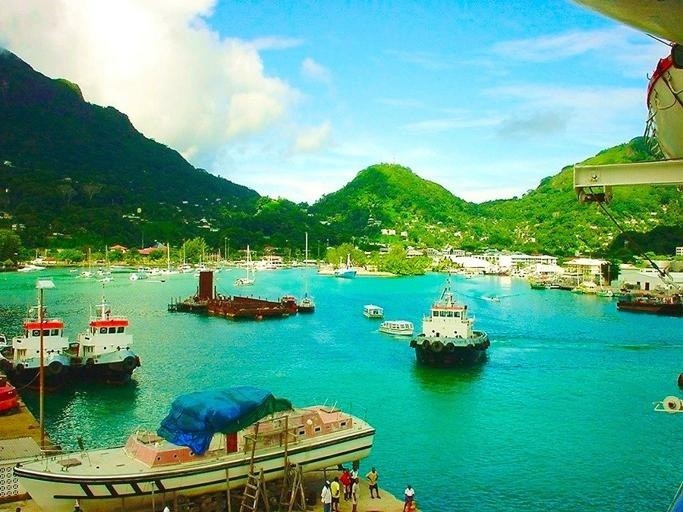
[240,467,269,512]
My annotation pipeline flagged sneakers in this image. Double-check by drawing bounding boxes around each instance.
[371,496,380,499]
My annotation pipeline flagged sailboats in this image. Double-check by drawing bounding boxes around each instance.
[68,239,282,287]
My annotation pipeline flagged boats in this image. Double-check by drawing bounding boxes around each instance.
[615,290,683,316]
[0,288,144,389]
[406,268,491,370]
[643,43,683,160]
[377,320,415,337]
[19,264,46,273]
[362,302,385,320]
[297,229,317,314]
[481,294,502,305]
[11,383,376,512]
[331,267,358,280]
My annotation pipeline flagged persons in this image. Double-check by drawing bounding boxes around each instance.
[403,485,414,512]
[320,466,360,511]
[162,504,174,512]
[73,504,83,512]
[16,507,21,512]
[364,467,381,498]
